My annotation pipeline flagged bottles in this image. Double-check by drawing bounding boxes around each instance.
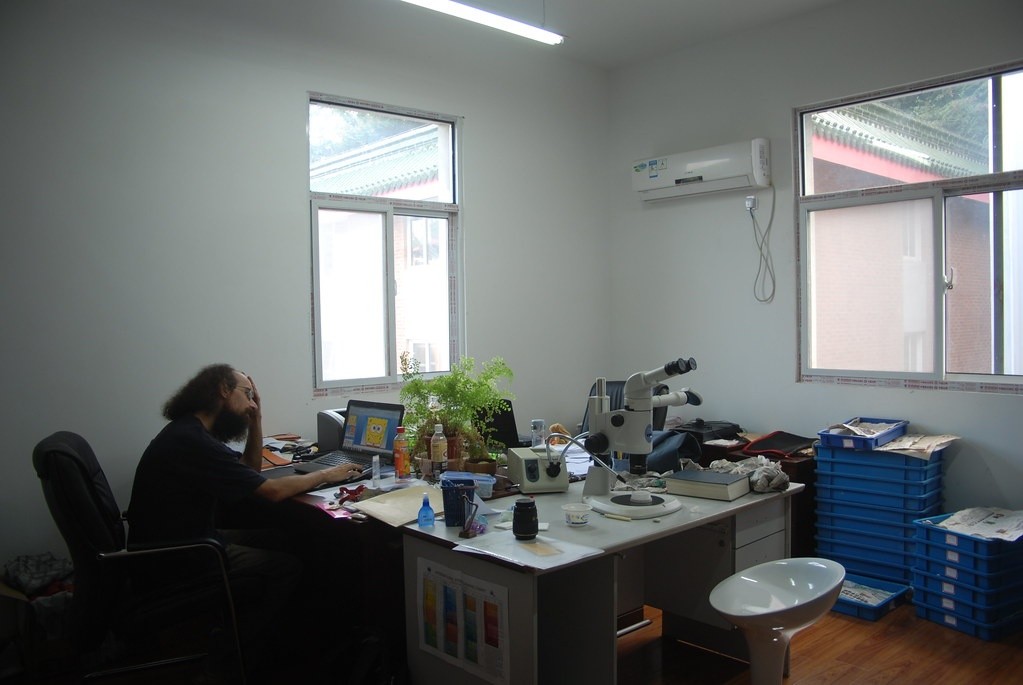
[428,423,449,486]
[417,492,435,528]
[393,426,411,485]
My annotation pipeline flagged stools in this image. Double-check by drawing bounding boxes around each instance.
[709,557,841,685]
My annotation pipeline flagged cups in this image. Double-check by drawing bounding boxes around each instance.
[531,419,546,447]
[512,498,538,541]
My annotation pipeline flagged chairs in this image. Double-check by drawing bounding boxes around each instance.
[33,432,248,683]
[580,380,666,436]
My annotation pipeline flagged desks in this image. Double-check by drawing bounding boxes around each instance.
[232,433,806,684]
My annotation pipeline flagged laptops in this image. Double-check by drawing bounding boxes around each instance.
[293,399,405,483]
[471,399,547,449]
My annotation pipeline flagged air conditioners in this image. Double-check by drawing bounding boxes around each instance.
[635,136,771,202]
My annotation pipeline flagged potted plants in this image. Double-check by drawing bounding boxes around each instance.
[393,351,516,476]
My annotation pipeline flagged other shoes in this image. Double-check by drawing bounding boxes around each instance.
[193,671,242,685]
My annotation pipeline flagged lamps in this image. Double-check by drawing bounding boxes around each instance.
[402,0,566,46]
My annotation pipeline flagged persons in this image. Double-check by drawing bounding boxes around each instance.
[127,364,362,685]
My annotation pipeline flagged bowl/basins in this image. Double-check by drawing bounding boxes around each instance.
[561,502,593,528]
[439,470,497,499]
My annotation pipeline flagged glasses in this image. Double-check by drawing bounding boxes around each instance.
[229,385,256,401]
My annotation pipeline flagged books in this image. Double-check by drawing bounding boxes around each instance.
[664,469,751,502]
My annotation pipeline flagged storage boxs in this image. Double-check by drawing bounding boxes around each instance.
[0,575,84,675]
[812,415,1023,642]
